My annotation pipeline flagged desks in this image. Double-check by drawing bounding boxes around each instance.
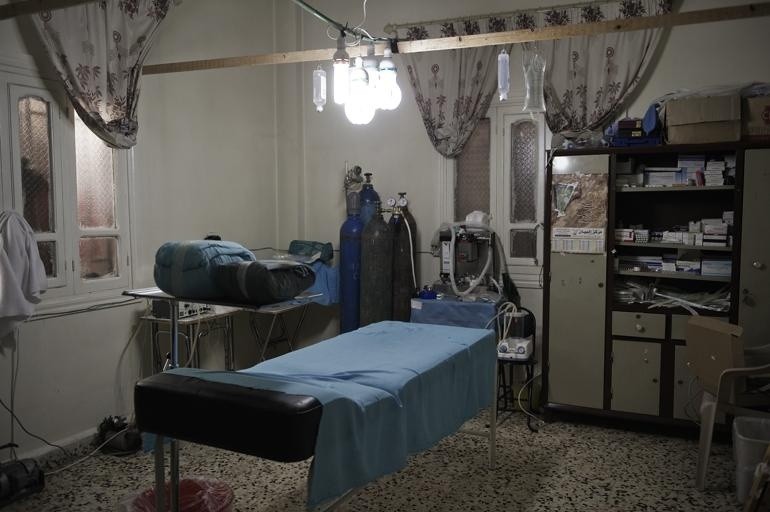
[250,299,328,361]
[135,299,242,372]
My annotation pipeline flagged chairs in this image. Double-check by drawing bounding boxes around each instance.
[486,306,539,434]
[697,340,769,492]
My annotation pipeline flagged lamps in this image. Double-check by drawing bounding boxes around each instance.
[331,29,403,127]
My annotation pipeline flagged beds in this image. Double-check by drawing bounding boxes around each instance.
[133,295,500,510]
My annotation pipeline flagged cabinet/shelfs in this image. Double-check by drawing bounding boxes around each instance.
[539,135,767,424]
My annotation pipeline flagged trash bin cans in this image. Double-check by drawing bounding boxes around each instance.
[732,416,770,504]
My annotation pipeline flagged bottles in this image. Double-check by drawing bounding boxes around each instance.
[312,69,327,112]
[497,53,511,104]
